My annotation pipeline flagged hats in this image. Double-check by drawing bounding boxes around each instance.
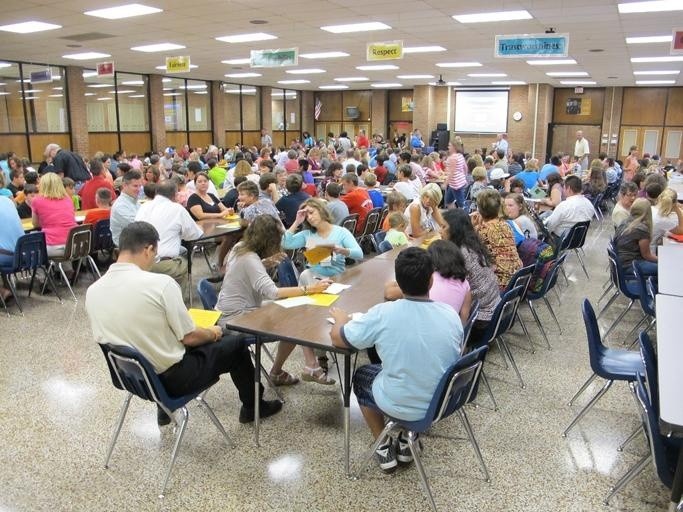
[490,169,510,180]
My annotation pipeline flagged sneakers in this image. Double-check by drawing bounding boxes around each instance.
[397,431,422,463]
[376,435,397,472]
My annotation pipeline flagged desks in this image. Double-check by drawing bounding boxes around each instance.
[370,220,503,261]
[220,259,408,478]
[655,243,681,295]
[182,214,246,307]
[648,294,683,440]
[17,206,117,297]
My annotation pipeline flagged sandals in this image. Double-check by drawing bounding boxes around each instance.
[0,289,13,307]
[268,371,299,387]
[67,272,77,287]
[301,366,336,385]
[318,357,329,375]
[207,272,225,283]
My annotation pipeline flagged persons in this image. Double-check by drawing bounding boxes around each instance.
[81,221,283,426]
[323,246,465,475]
[1,127,683,388]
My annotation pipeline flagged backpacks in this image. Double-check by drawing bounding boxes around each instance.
[517,229,557,295]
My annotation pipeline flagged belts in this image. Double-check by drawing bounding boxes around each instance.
[161,256,170,260]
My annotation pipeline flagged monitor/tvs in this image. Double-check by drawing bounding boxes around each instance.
[437,123,447,132]
[345,106,361,118]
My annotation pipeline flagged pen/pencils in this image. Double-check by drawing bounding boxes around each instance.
[313,277,332,284]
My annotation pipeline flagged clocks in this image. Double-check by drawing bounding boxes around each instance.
[512,111,522,122]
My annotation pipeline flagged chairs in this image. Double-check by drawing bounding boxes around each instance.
[0,156,682,510]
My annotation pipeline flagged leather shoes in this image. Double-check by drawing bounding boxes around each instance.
[156,408,171,425]
[239,400,283,423]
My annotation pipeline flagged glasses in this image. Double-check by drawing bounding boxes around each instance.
[154,250,160,264]
